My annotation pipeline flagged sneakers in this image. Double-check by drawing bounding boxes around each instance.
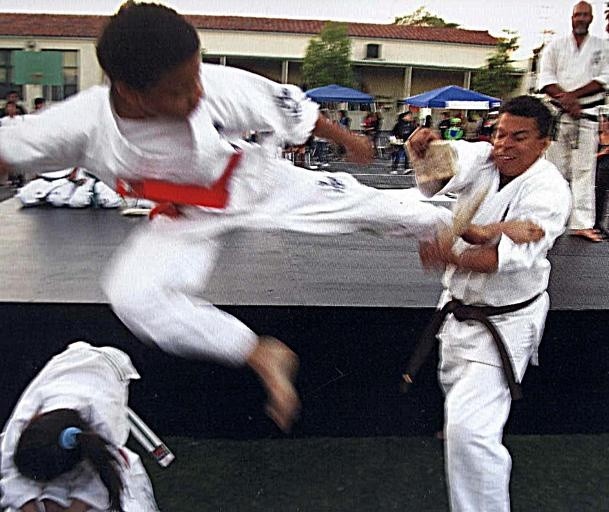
[403,169,413,175]
[390,171,398,175]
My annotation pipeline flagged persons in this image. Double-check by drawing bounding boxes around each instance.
[406,95,575,511]
[0,341,177,512]
[0,0,546,429]
[0,91,608,215]
[533,0,609,244]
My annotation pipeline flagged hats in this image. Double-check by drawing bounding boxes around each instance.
[450,118,461,124]
[488,111,499,115]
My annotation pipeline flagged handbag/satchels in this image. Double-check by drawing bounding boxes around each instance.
[388,128,404,145]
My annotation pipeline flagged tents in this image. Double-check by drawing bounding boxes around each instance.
[408,85,502,109]
[303,84,372,104]
